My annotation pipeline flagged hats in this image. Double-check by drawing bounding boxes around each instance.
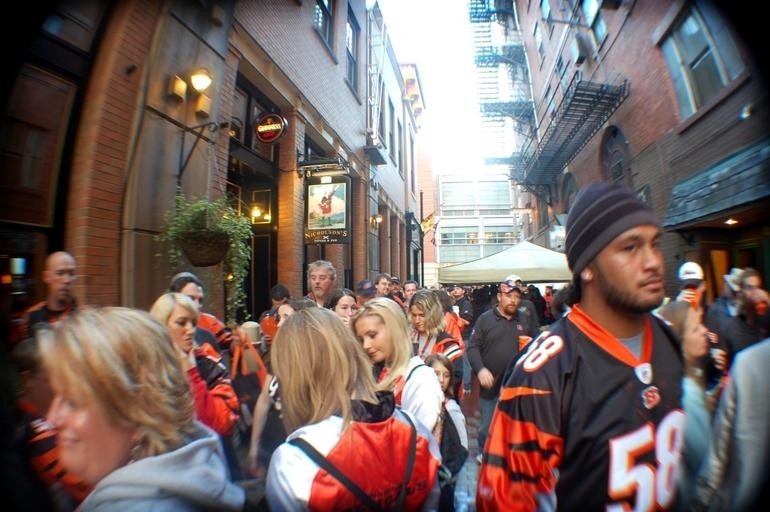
[357,267,401,295]
[677,259,705,288]
[497,274,526,295]
[564,178,663,284]
[722,267,746,293]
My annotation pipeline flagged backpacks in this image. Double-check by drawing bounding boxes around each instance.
[402,362,469,488]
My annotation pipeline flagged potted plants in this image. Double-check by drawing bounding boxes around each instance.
[150,191,258,326]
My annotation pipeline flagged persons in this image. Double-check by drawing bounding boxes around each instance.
[477,183,688,511]
[1,252,770,512]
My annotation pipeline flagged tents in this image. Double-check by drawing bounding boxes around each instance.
[438,240,573,289]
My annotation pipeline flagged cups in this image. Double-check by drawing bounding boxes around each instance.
[687,287,702,309]
[749,293,767,315]
[518,336,530,352]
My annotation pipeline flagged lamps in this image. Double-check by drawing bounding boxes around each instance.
[246,201,265,217]
[372,211,384,225]
[178,61,216,190]
